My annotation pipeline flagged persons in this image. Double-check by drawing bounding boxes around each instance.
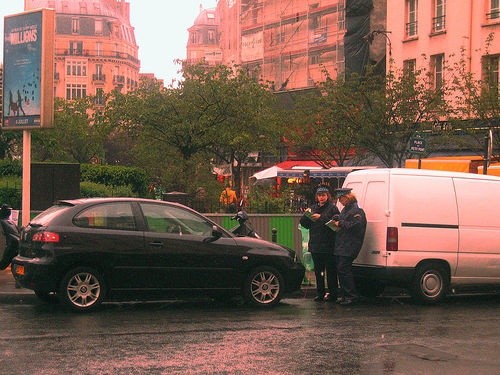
[298,170,314,186]
[220,182,238,205]
[324,187,367,307]
[299,183,342,302]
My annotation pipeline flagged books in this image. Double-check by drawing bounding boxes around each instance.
[304,207,317,223]
[325,220,340,232]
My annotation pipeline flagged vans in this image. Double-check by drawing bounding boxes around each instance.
[332,167,499,305]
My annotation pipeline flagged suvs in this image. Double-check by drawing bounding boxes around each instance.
[10,196,307,312]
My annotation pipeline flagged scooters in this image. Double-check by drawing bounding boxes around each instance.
[229,199,261,239]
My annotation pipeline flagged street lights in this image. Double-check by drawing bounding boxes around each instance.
[361,29,392,158]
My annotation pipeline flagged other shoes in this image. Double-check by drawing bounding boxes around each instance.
[326,296,334,302]
[338,299,353,306]
[314,296,323,301]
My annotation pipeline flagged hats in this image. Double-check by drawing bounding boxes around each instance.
[315,184,331,193]
[334,188,353,198]
